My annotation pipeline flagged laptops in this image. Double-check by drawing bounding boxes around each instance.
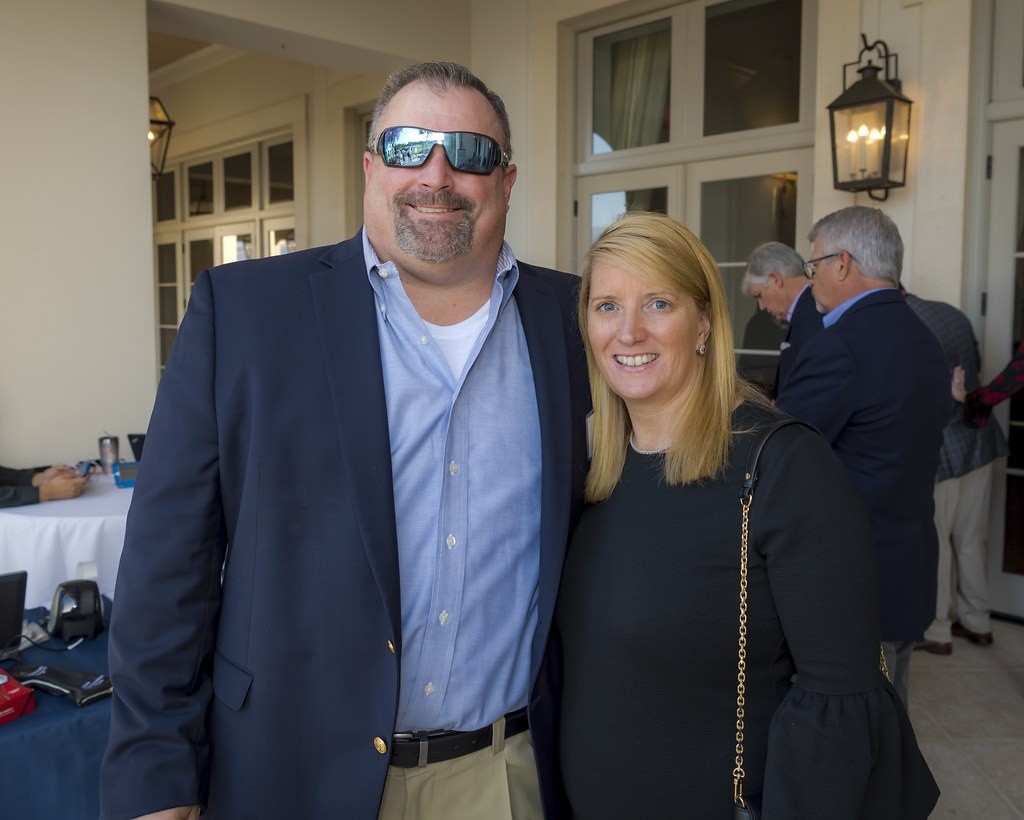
[127,434,145,461]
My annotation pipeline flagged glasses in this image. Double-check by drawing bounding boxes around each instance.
[802,250,856,280]
[368,126,509,176]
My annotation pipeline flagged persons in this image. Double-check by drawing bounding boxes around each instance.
[102,62,584,820]
[742,206,1024,653]
[0,465,90,508]
[531,215,941,820]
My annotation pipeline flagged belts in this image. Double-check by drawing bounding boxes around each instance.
[388,707,529,769]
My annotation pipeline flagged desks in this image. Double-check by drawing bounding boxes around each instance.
[0,473,135,611]
[0,590,114,820]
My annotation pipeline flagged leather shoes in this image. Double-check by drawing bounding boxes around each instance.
[913,642,952,655]
[952,620,993,646]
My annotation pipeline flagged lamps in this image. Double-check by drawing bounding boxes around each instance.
[826,31,913,200]
[150,95,176,180]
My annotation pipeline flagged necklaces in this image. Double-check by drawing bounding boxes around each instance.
[630,430,672,455]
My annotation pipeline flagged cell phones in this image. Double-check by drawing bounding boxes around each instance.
[72,462,89,479]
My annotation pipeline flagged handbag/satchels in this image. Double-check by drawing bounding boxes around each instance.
[735,418,895,820]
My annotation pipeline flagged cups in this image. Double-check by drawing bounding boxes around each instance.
[100,437,118,475]
